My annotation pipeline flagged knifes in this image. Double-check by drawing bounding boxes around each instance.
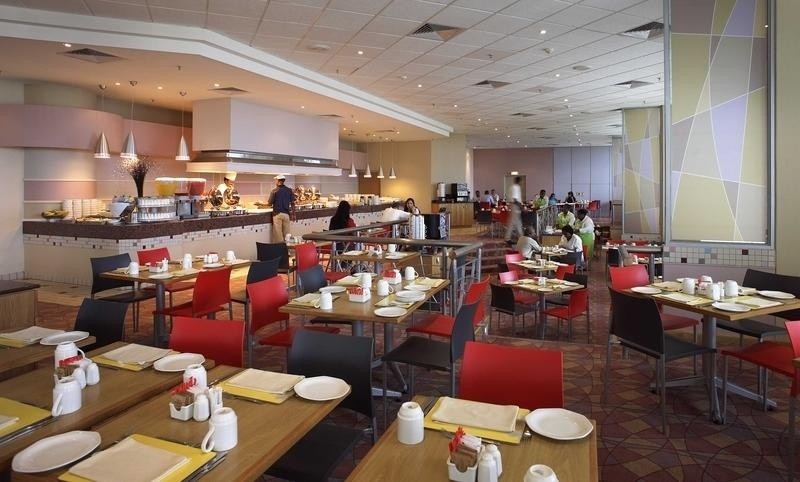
[223,392,265,405]
[182,451,228,482]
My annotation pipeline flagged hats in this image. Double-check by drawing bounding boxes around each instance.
[225,172,236,181]
[274,175,285,179]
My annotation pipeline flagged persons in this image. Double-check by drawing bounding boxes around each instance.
[492,189,499,201]
[475,190,482,202]
[217,173,237,195]
[575,208,596,242]
[504,177,525,246]
[404,198,420,215]
[379,202,411,236]
[261,175,296,243]
[517,225,542,274]
[329,200,356,268]
[548,225,584,277]
[558,203,576,229]
[548,193,557,205]
[483,190,494,203]
[534,189,546,209]
[565,191,577,203]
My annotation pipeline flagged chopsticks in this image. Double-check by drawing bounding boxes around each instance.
[0,417,57,445]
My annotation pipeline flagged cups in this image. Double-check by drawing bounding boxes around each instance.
[695,281,708,295]
[376,279,393,296]
[724,280,744,297]
[137,211,178,221]
[699,275,714,283]
[225,250,236,261]
[314,291,333,309]
[357,273,373,288]
[54,341,86,370]
[180,253,192,269]
[137,194,175,206]
[397,401,424,445]
[706,284,721,301]
[387,243,397,252]
[524,463,559,482]
[182,364,208,398]
[404,265,418,281]
[677,278,695,295]
[125,261,140,275]
[51,377,82,417]
[200,406,238,453]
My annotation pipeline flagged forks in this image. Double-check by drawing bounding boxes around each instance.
[523,427,533,443]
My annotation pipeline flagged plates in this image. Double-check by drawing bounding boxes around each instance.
[39,330,89,345]
[149,273,174,280]
[711,302,751,312]
[676,277,699,285]
[73,199,82,218]
[153,352,205,372]
[90,198,103,215]
[396,289,425,298]
[631,286,662,295]
[202,264,225,268]
[385,255,403,260]
[403,284,431,291]
[82,199,91,217]
[525,406,596,440]
[195,256,204,259]
[374,306,407,317]
[563,281,579,286]
[539,288,552,292]
[352,272,378,277]
[756,290,796,299]
[293,375,350,401]
[11,429,101,474]
[62,198,74,218]
[319,285,347,293]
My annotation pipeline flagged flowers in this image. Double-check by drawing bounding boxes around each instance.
[110,154,158,197]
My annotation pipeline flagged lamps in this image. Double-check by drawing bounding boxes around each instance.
[94,83,110,161]
[346,129,357,180]
[389,139,397,179]
[119,80,140,160]
[172,91,191,162]
[364,132,372,178]
[376,133,385,180]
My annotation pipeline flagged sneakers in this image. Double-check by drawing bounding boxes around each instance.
[504,240,513,244]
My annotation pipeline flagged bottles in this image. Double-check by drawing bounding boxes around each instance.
[718,282,724,296]
[204,254,207,263]
[72,367,87,390]
[486,443,503,477]
[208,255,212,264]
[209,386,223,415]
[538,278,545,285]
[540,259,546,267]
[86,362,100,385]
[162,257,169,272]
[193,393,210,422]
[476,452,498,482]
